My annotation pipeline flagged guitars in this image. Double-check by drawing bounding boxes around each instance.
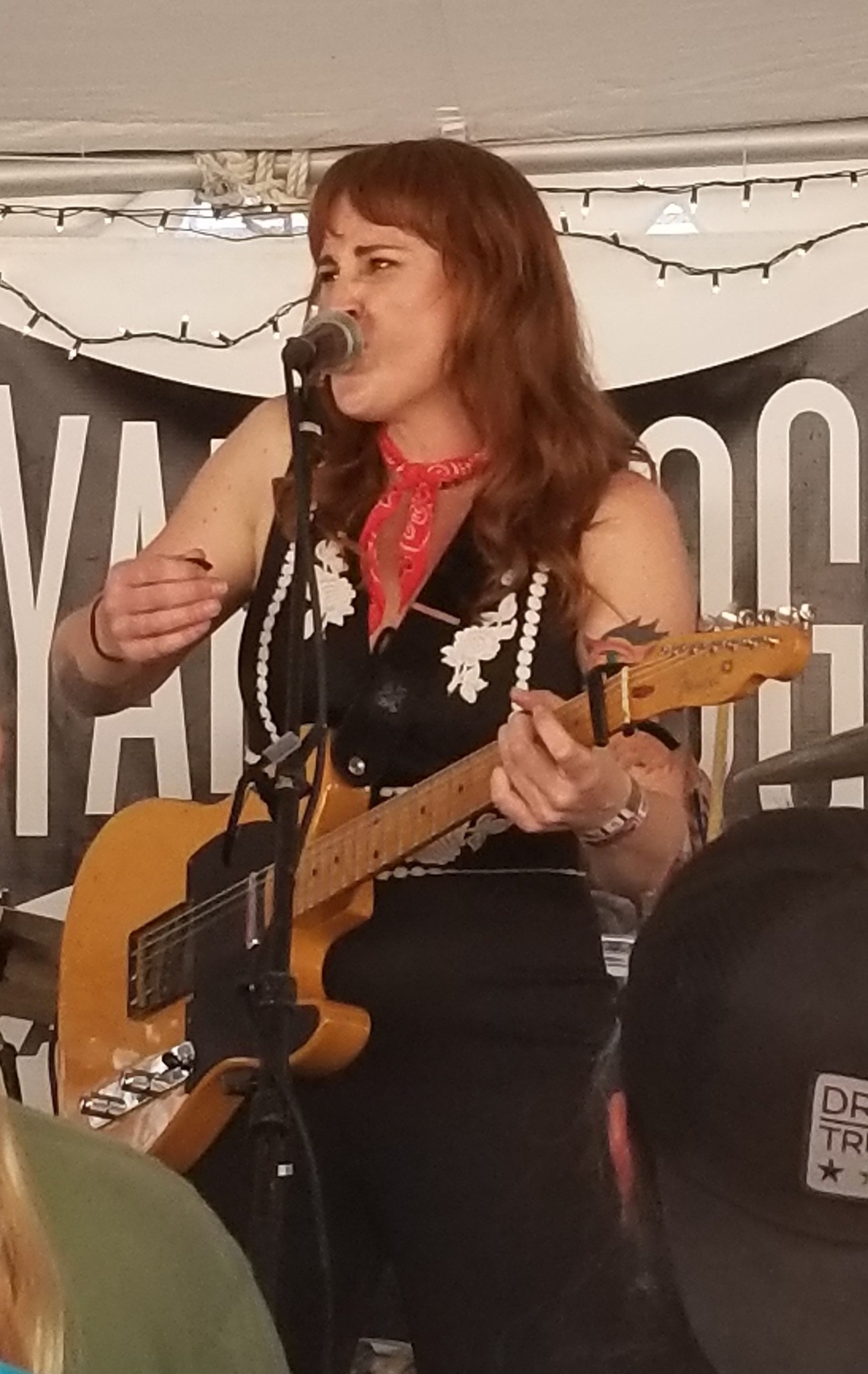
[55,597,818,1176]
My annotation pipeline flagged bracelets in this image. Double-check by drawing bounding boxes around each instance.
[90,596,123,662]
[581,773,649,850]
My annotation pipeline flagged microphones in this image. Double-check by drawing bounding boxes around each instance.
[280,311,366,375]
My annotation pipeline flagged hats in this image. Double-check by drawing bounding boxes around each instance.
[625,801,868,1374]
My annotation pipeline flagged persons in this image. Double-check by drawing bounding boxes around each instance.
[0,1096,82,1374]
[50,138,696,1374]
[593,808,868,1374]
